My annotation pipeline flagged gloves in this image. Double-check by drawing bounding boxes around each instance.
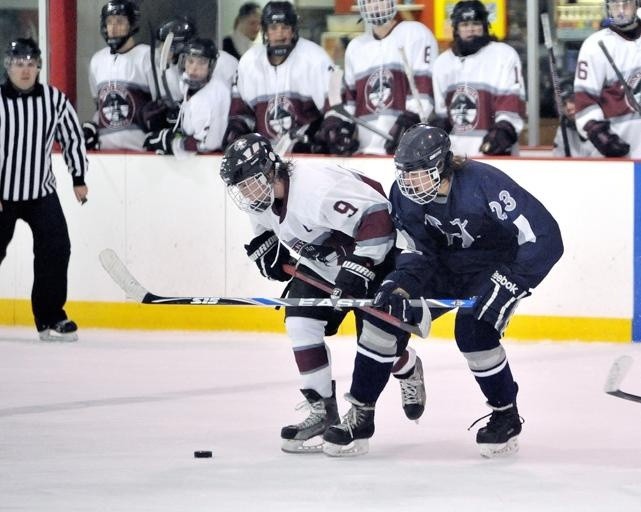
[375,266,422,333]
[383,112,421,155]
[478,119,519,157]
[474,264,533,335]
[332,253,378,301]
[139,125,174,156]
[222,114,251,147]
[583,117,631,158]
[80,119,101,150]
[314,107,358,155]
[243,229,298,282]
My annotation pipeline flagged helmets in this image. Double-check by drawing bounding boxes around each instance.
[259,1,301,57]
[98,0,142,55]
[449,1,491,56]
[358,0,397,29]
[217,131,281,221]
[390,122,456,205]
[3,35,41,95]
[175,38,218,92]
[597,0,641,30]
[158,17,197,51]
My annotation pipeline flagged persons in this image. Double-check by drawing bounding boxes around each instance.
[222,3,262,60]
[159,23,238,107]
[344,2,438,154]
[323,122,564,458]
[143,40,233,153]
[83,3,154,152]
[1,38,88,343]
[574,1,641,157]
[238,2,342,147]
[553,80,578,161]
[435,1,528,155]
[219,134,426,452]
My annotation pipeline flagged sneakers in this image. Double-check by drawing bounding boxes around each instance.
[279,378,342,441]
[320,390,375,446]
[476,393,523,443]
[36,318,77,335]
[392,349,428,423]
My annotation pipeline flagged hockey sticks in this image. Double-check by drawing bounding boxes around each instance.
[539,12,572,157]
[280,264,431,339]
[597,40,639,112]
[328,65,395,143]
[97,247,485,309]
[397,45,429,126]
[158,31,178,108]
